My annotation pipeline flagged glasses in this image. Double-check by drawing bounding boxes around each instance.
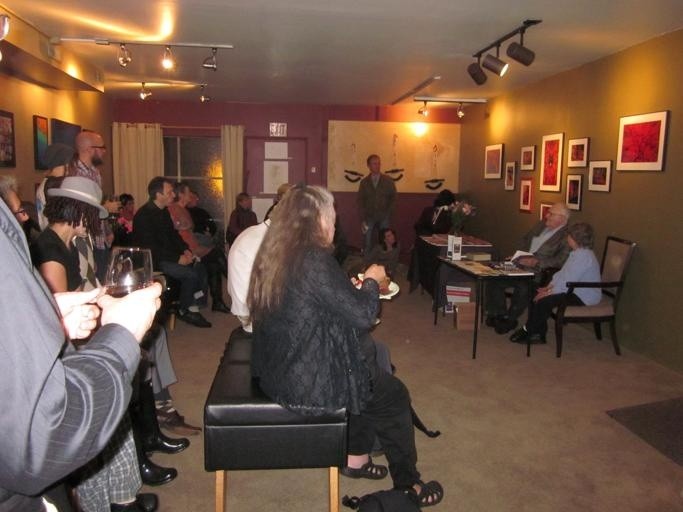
[11,208,27,217]
[90,144,106,150]
[547,210,564,217]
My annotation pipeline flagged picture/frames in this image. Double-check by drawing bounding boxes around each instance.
[616,110,670,172]
[519,175,535,214]
[504,162,516,191]
[589,159,612,192]
[520,144,536,171]
[540,131,564,193]
[0,110,16,168]
[564,173,583,212]
[567,137,591,169]
[538,199,555,220]
[33,115,49,170]
[484,144,505,181]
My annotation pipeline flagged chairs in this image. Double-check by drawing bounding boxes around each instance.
[542,234,639,357]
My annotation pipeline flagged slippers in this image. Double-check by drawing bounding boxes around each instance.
[342,455,388,480]
[406,479,444,507]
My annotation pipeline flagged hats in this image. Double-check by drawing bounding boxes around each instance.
[47,176,109,219]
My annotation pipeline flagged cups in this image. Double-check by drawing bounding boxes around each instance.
[105,246,153,298]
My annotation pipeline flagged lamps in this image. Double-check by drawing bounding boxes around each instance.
[455,103,465,119]
[481,42,509,77]
[201,48,218,71]
[418,100,432,117]
[138,80,153,102]
[118,44,132,68]
[464,54,488,87]
[199,85,214,105]
[506,31,536,66]
[159,46,175,69]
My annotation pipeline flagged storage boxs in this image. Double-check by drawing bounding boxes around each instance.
[443,283,473,314]
[454,302,481,333]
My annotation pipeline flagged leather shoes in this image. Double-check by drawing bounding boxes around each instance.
[177,308,212,329]
[140,427,190,457]
[109,492,159,511]
[528,324,547,344]
[155,416,202,437]
[139,456,179,487]
[211,302,231,314]
[485,316,503,326]
[510,327,528,343]
[495,319,518,334]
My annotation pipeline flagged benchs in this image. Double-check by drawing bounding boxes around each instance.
[203,325,348,511]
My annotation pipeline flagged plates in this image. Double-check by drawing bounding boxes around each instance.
[354,280,400,300]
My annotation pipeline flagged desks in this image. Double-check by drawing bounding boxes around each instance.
[418,233,494,299]
[433,260,536,361]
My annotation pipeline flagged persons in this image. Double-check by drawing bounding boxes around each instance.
[226,193,258,248]
[117,193,134,234]
[167,183,230,313]
[486,203,570,334]
[73,131,123,285]
[131,177,212,329]
[358,155,397,253]
[343,229,400,282]
[29,176,189,487]
[416,190,458,314]
[245,185,443,508]
[510,223,602,343]
[226,182,300,334]
[35,143,96,291]
[185,192,217,239]
[42,176,202,434]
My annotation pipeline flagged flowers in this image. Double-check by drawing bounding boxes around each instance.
[448,201,482,237]
